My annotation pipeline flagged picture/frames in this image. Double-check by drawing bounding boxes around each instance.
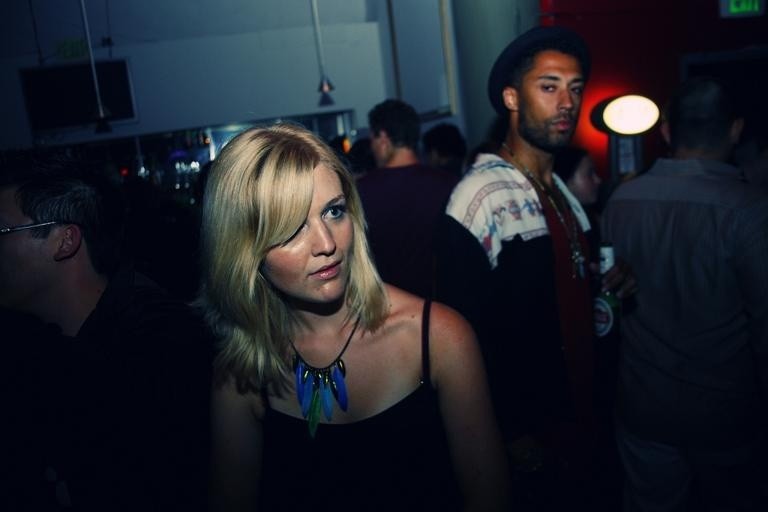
[387,0,456,125]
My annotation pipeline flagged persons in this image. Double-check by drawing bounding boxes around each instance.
[1,162,218,510]
[346,98,502,296]
[197,120,501,511]
[438,27,640,511]
[555,74,768,512]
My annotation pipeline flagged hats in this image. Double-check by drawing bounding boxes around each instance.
[488,25,592,117]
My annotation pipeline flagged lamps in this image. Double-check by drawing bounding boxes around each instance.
[311,1,335,92]
[81,1,114,133]
[589,92,661,199]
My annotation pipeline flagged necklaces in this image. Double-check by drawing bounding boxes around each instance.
[289,315,361,438]
[501,140,584,279]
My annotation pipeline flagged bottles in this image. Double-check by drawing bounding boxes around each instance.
[592,247,623,370]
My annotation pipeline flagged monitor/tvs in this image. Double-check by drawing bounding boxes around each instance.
[16,56,136,134]
